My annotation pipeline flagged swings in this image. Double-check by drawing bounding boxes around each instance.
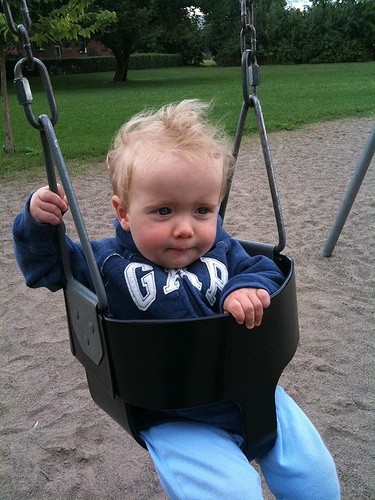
[2,0,300,462]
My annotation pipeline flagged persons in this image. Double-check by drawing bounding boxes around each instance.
[12,98,341,500]
